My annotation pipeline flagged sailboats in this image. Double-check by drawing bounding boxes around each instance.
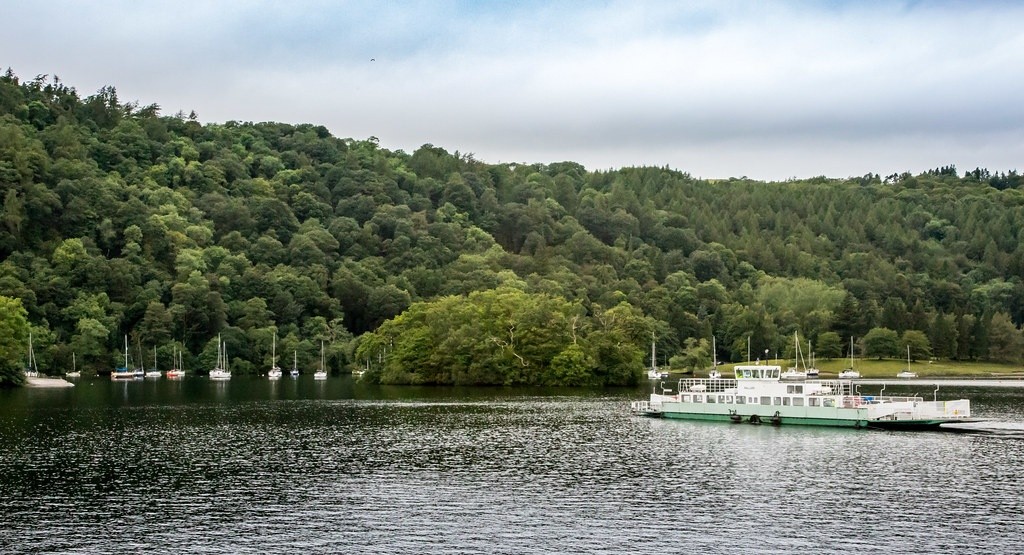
[21,332,81,386]
[647,331,669,379]
[167,347,185,378]
[289,351,299,378]
[781,330,820,378]
[110,335,162,379]
[314,341,328,381]
[209,333,232,382]
[896,345,919,378]
[837,335,860,379]
[709,337,723,378]
[268,332,282,378]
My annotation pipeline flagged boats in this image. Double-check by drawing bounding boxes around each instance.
[650,334,971,430]
[630,401,662,417]
